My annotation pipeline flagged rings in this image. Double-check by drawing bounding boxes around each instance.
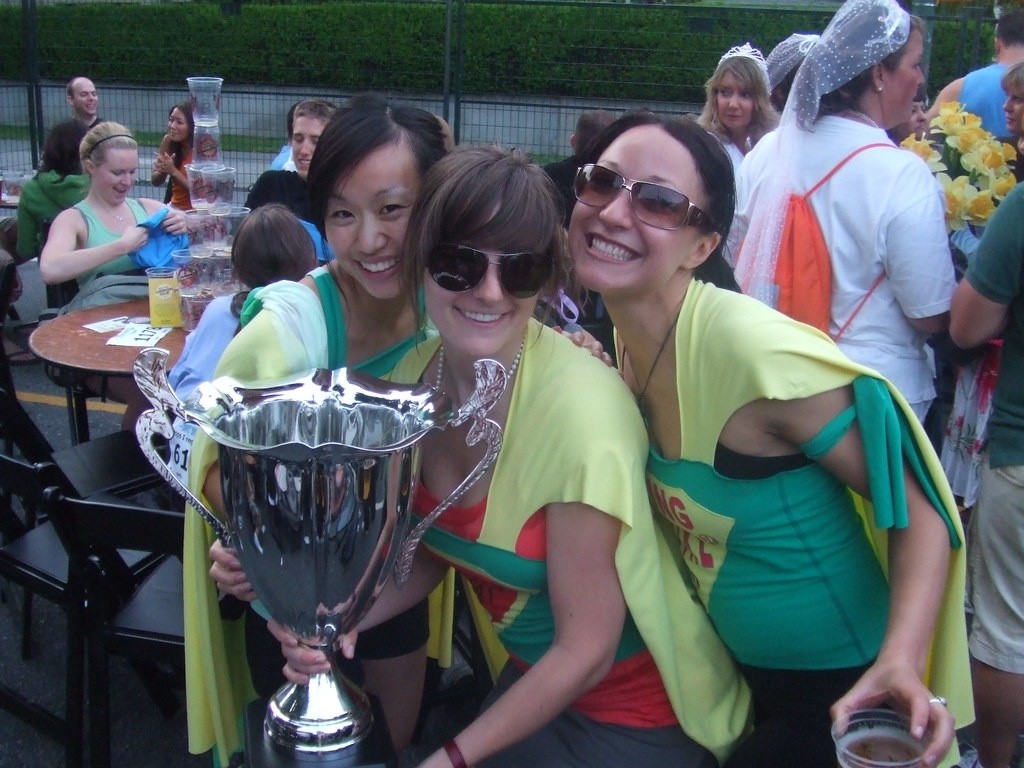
[929,695,948,710]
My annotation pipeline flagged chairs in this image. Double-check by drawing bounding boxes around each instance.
[0,248,16,325]
[0,342,161,662]
[41,486,185,768]
[0,451,166,768]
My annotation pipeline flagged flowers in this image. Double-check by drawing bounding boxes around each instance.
[899,101,1018,233]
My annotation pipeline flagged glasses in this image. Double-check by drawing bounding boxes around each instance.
[574,164,715,233]
[426,241,553,298]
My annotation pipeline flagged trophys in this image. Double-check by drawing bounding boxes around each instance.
[131,348,507,768]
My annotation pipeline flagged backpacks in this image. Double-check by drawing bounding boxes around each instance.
[736,125,898,341]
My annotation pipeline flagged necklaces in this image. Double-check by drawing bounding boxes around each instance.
[620,308,679,410]
[839,106,876,126]
[100,203,126,222]
[432,335,526,415]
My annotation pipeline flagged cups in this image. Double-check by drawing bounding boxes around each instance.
[146,266,173,281]
[831,710,932,768]
[203,167,238,217]
[174,208,253,334]
[185,163,217,211]
[187,77,224,127]
[0,171,37,205]
[190,125,227,171]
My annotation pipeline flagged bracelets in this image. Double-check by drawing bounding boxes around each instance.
[351,593,363,613]
[440,739,468,768]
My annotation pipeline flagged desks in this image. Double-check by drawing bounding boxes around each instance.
[28,298,192,448]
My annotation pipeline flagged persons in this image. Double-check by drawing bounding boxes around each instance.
[568,109,1024,768]
[239,451,400,624]
[181,97,617,768]
[208,139,760,768]
[0,76,343,432]
[696,0,1024,474]
